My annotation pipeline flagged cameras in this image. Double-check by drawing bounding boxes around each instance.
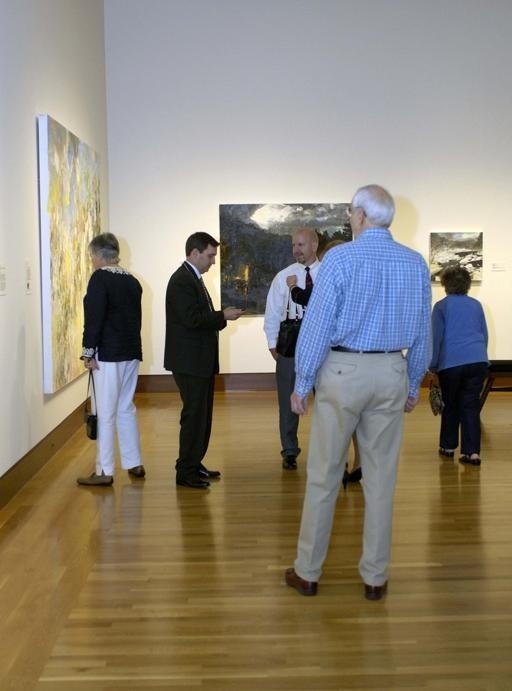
[241,307,246,311]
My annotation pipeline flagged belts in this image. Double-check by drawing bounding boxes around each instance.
[331,344,400,354]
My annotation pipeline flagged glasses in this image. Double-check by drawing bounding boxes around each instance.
[346,206,368,219]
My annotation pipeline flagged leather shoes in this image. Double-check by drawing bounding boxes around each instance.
[349,467,361,482]
[343,470,349,489]
[77,472,113,485]
[199,463,220,479]
[282,455,297,470]
[365,580,387,600]
[128,466,145,477]
[285,568,317,595]
[176,476,209,487]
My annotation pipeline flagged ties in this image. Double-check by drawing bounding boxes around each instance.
[201,276,212,314]
[305,266,313,289]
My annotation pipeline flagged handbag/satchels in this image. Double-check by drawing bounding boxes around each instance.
[429,380,444,417]
[275,319,303,356]
[86,415,97,439]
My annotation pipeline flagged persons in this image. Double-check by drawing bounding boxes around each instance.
[74,231,146,488]
[264,226,322,470]
[286,240,362,487]
[162,233,246,488]
[428,265,489,466]
[283,186,435,604]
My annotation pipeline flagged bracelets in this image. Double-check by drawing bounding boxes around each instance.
[289,284,296,289]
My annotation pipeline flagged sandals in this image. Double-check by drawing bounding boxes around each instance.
[439,448,454,457]
[459,453,480,466]
